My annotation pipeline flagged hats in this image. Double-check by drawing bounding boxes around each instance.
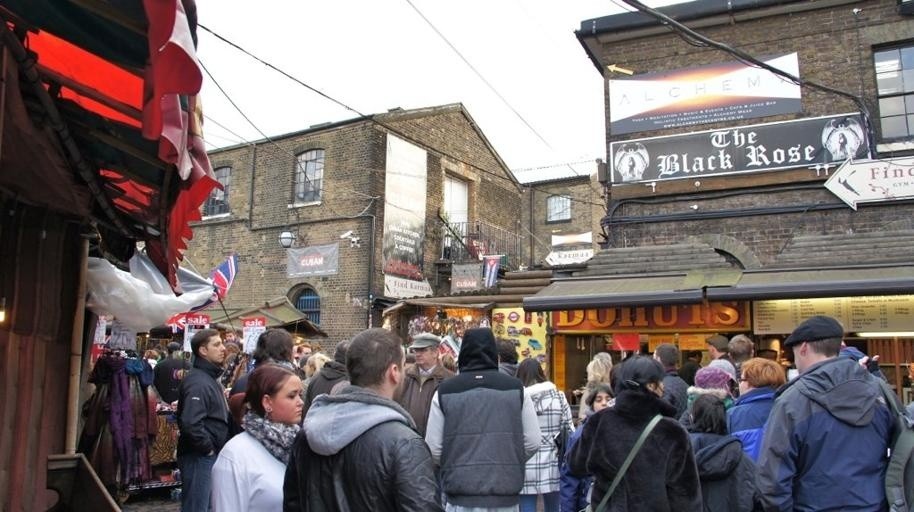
[781,315,847,349]
[165,341,181,352]
[695,365,732,392]
[703,334,729,353]
[407,333,441,349]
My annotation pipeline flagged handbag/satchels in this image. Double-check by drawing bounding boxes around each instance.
[553,389,574,474]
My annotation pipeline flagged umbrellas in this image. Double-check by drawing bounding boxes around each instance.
[182,253,239,313]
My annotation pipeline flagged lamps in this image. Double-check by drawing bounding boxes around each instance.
[279,207,307,248]
